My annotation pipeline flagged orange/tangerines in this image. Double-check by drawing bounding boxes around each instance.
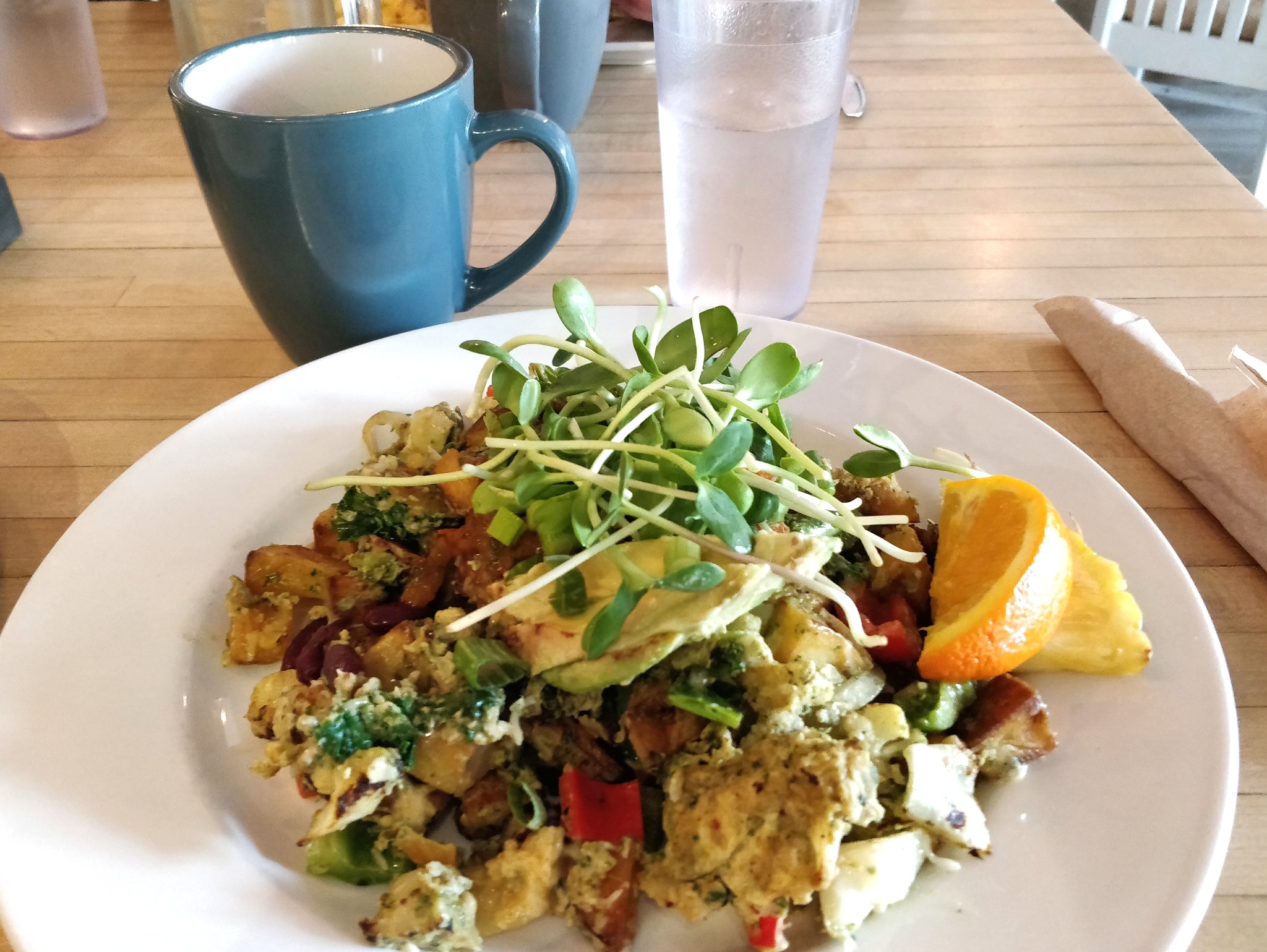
[917,473,1074,683]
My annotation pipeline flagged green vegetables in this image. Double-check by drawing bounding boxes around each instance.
[299,276,992,888]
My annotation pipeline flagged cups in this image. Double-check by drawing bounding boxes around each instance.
[426,0,610,133]
[0,0,110,140]
[650,0,860,321]
[166,25,579,367]
[168,0,384,63]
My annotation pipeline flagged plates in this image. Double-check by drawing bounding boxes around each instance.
[0,305,1240,952]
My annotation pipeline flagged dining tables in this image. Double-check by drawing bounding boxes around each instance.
[0,0,1267,952]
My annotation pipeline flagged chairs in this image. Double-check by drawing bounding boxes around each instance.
[1090,0,1267,208]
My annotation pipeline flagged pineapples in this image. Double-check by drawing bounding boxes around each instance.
[1011,527,1153,676]
